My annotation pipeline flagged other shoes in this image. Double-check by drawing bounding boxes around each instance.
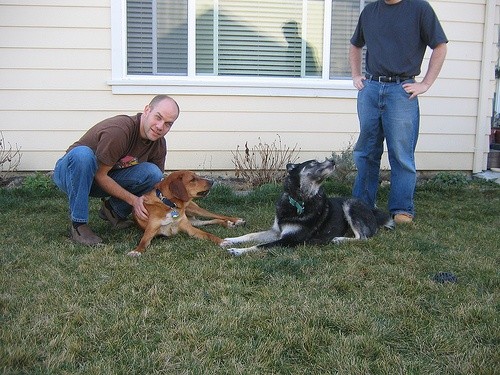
[70,223,105,246]
[98,197,135,230]
[393,213,413,224]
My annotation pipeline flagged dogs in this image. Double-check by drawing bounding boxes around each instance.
[221,158,396,258]
[126,170,246,258]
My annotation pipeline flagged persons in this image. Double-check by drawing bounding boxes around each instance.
[350,0,449,228]
[53,95,179,246]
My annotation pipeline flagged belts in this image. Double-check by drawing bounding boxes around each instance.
[365,73,415,82]
